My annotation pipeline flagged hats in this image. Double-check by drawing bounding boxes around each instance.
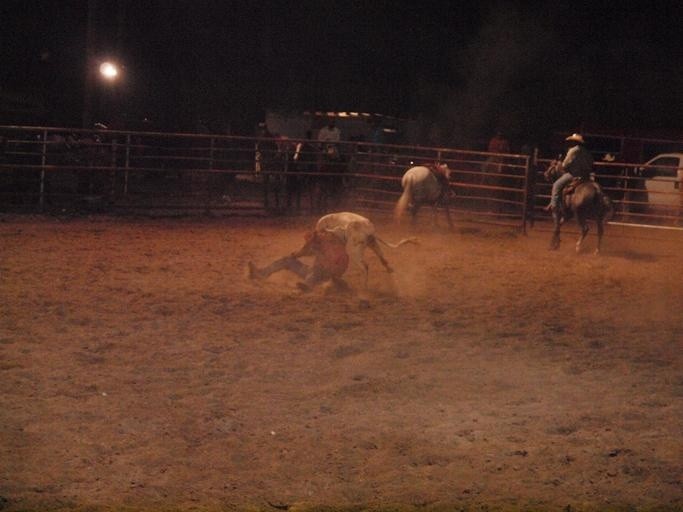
[565,133,584,143]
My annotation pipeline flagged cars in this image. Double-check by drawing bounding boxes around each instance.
[633,152,683,210]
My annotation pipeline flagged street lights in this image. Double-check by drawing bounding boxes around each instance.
[83,63,118,130]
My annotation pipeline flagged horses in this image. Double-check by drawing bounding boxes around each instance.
[543,159,611,257]
[257,138,306,210]
[306,139,344,209]
[394,163,455,230]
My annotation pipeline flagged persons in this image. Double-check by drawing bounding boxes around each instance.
[242,227,351,296]
[262,110,626,214]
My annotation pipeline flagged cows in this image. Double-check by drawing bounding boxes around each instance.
[310,211,420,285]
